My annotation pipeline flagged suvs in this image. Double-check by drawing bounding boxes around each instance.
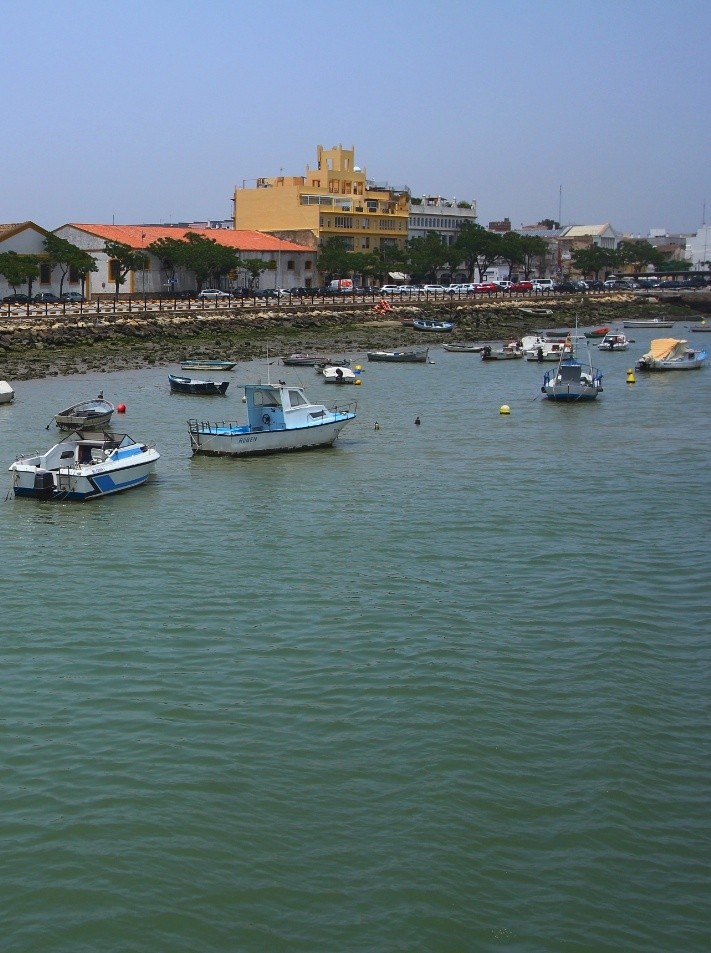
[377,284,424,296]
[424,283,496,296]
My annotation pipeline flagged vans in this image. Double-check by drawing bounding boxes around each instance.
[493,281,514,290]
[328,279,354,292]
[531,279,555,291]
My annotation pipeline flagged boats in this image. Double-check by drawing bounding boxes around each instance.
[443,313,606,400]
[518,307,553,317]
[635,338,707,370]
[584,327,630,351]
[367,347,429,362]
[623,320,675,328]
[683,325,711,333]
[167,342,357,455]
[402,317,455,331]
[9,428,161,502]
[55,390,115,431]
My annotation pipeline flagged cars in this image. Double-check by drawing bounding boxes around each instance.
[605,277,708,294]
[552,280,605,294]
[505,283,533,292]
[175,287,379,300]
[3,292,87,304]
